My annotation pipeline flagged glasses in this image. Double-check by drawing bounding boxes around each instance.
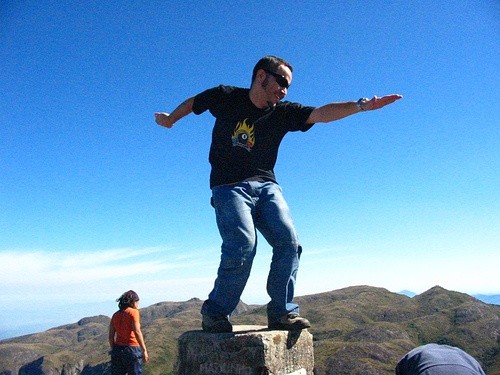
[265,70,291,90]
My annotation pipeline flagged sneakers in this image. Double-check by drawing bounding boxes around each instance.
[266,310,310,332]
[200,310,234,334]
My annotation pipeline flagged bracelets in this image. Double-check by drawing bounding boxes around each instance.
[355,97,364,112]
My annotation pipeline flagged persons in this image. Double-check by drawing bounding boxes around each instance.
[108,289,148,375]
[151,54,405,331]
[396,342,486,375]
